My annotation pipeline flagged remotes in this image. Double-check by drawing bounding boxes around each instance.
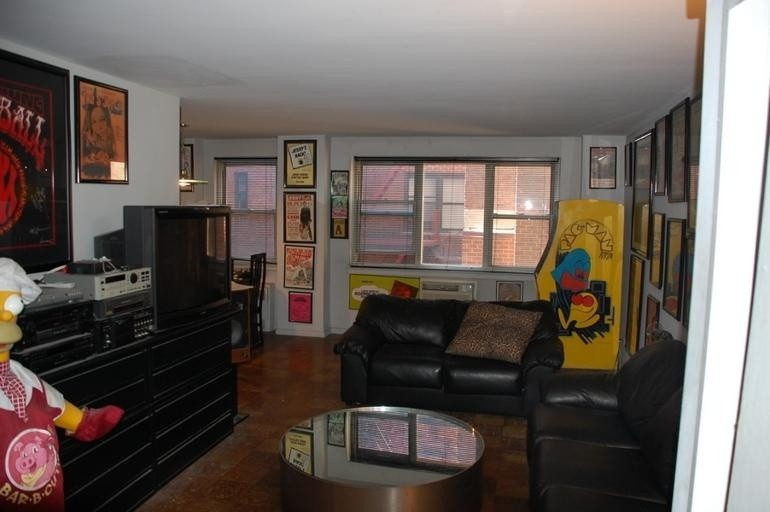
[38,283,74,289]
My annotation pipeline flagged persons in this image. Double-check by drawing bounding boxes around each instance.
[84,106,113,159]
[0,255,123,512]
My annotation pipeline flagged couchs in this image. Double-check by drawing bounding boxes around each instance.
[523,341,686,512]
[333,293,564,418]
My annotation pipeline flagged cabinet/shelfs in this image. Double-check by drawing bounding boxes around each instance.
[37,300,244,512]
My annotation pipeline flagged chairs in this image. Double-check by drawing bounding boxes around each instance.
[234,251,267,358]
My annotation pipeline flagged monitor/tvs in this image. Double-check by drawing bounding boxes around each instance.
[95,206,231,332]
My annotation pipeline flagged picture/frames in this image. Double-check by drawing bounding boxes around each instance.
[495,280,524,302]
[282,138,318,188]
[288,291,313,324]
[282,417,315,477]
[348,272,421,310]
[0,48,73,274]
[326,411,346,448]
[72,74,130,185]
[283,245,316,291]
[330,170,350,240]
[179,144,194,193]
[419,277,478,303]
[283,191,318,244]
[589,90,703,357]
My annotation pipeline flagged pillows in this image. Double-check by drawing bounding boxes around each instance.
[443,300,544,365]
